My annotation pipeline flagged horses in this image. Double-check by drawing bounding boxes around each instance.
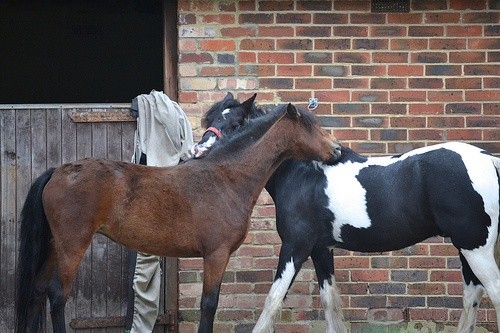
[13,103,342,333]
[191,91,500,333]
